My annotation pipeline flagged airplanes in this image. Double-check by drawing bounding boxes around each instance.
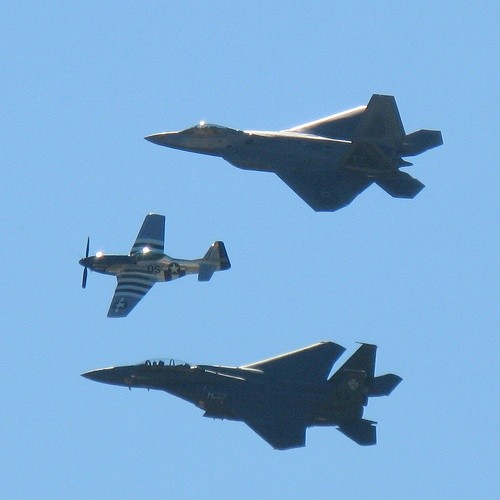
[77,212,233,319]
[78,340,404,452]
[143,93,444,213]
